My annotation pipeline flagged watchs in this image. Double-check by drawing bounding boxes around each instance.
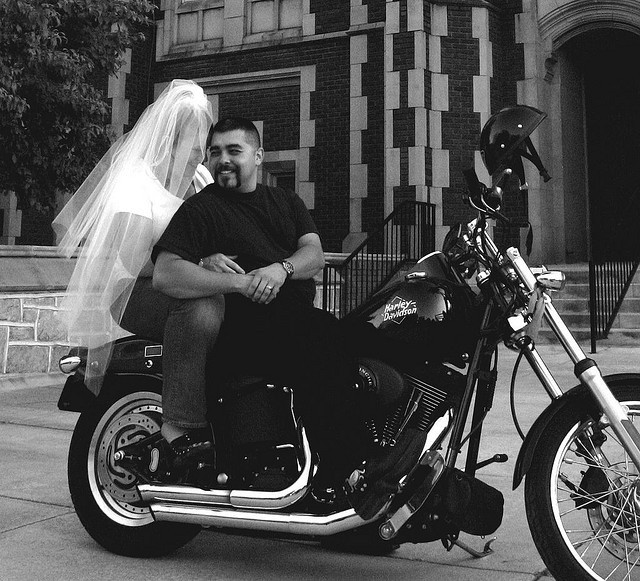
[276,259,294,281]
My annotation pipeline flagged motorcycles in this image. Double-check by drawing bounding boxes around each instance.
[56,104,640,581]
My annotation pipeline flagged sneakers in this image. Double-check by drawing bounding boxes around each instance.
[343,463,386,520]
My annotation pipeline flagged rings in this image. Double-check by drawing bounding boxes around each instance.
[266,286,273,290]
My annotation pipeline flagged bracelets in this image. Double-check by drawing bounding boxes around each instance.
[199,258,204,269]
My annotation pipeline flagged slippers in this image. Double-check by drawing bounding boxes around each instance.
[163,431,211,458]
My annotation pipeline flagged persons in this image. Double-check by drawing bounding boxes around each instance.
[152,118,427,520]
[51,80,246,459]
